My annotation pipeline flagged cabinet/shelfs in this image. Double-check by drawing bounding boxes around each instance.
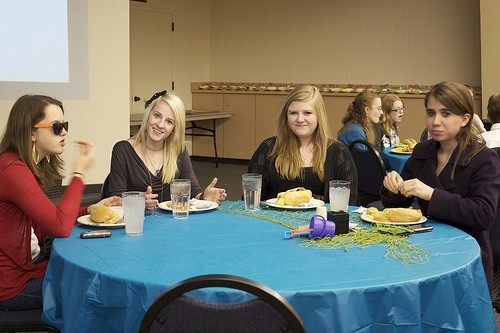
[130,5,174,114]
[191,83,482,168]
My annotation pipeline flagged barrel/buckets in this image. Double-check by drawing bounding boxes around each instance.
[309,215,336,240]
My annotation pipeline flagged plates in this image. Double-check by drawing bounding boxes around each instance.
[159,200,218,212]
[361,211,427,225]
[76,211,145,229]
[391,147,413,154]
[266,197,325,209]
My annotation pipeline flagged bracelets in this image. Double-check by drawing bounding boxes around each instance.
[74,171,88,179]
[199,192,204,200]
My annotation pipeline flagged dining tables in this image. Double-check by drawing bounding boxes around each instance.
[380,144,416,176]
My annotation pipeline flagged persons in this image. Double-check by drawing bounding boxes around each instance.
[100,89,228,213]
[382,93,406,151]
[381,82,499,309]
[336,90,395,209]
[242,84,357,205]
[0,93,122,333]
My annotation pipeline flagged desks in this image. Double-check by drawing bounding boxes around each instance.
[130,108,228,169]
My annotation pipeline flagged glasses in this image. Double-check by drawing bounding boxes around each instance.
[391,106,405,113]
[32,119,69,136]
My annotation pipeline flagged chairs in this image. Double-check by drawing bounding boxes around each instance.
[41,197,497,333]
[138,274,307,333]
[0,307,59,333]
[347,139,387,203]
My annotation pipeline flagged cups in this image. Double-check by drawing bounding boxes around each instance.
[170,179,191,219]
[329,180,351,213]
[243,173,262,211]
[121,191,146,236]
